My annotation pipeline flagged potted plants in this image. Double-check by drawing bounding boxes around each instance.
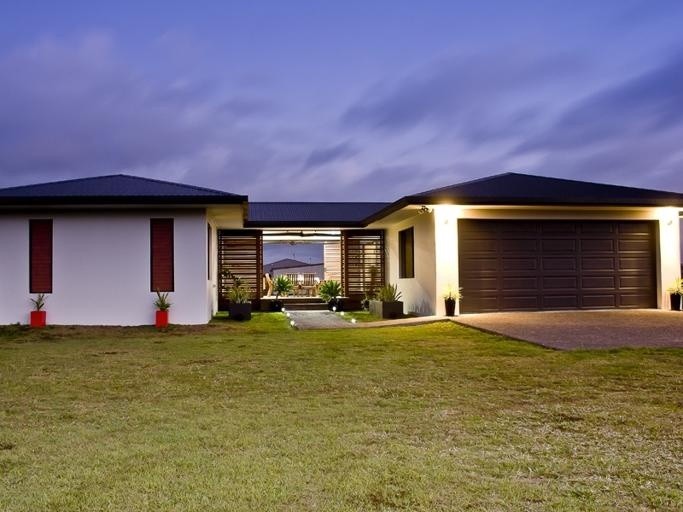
[439,280,465,317]
[317,279,344,311]
[368,282,404,318]
[222,267,252,321]
[269,273,295,311]
[27,291,51,327]
[665,274,683,310]
[152,287,174,328]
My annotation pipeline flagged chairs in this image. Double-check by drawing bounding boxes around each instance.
[286,272,317,296]
